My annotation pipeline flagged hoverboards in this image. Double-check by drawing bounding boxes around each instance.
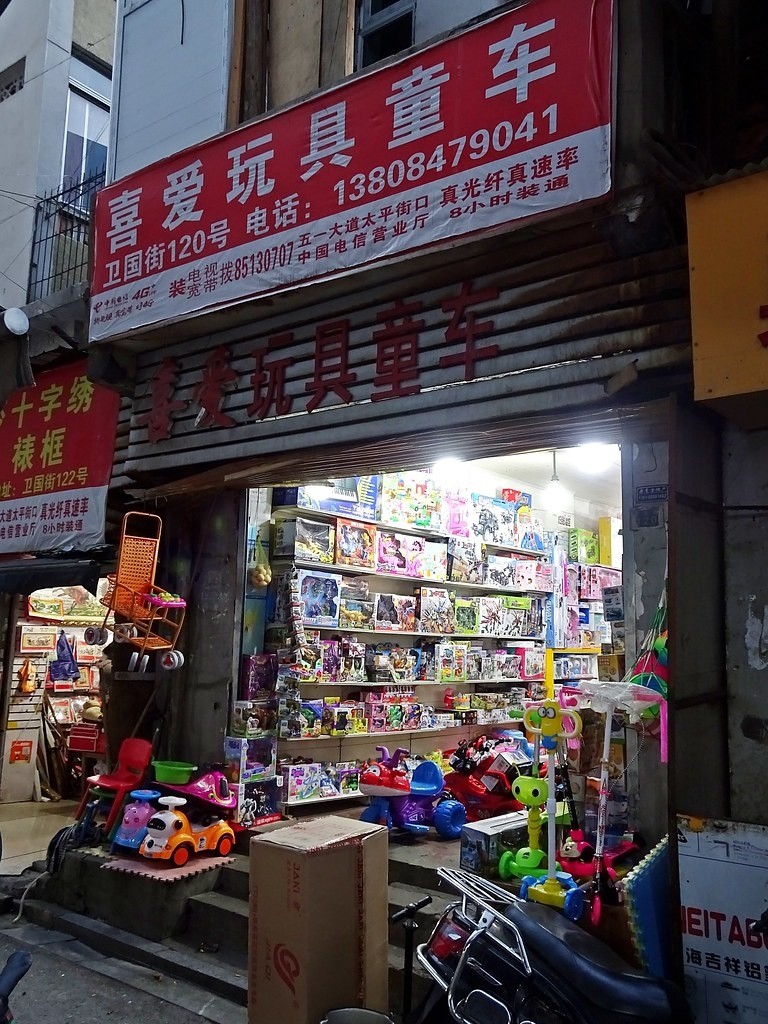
[499,682,664,925]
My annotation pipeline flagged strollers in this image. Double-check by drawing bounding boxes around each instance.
[84,512,186,675]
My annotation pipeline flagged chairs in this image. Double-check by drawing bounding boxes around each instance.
[74,738,155,840]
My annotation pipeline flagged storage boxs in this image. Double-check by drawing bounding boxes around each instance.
[597,514,622,571]
[248,816,387,1024]
[568,528,600,562]
[276,470,548,803]
[547,531,569,565]
[460,811,548,877]
[559,584,628,828]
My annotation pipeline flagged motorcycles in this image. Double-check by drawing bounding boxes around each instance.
[414,865,671,1024]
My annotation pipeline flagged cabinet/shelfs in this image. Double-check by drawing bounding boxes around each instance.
[50,615,115,732]
[267,507,547,806]
[553,563,619,680]
